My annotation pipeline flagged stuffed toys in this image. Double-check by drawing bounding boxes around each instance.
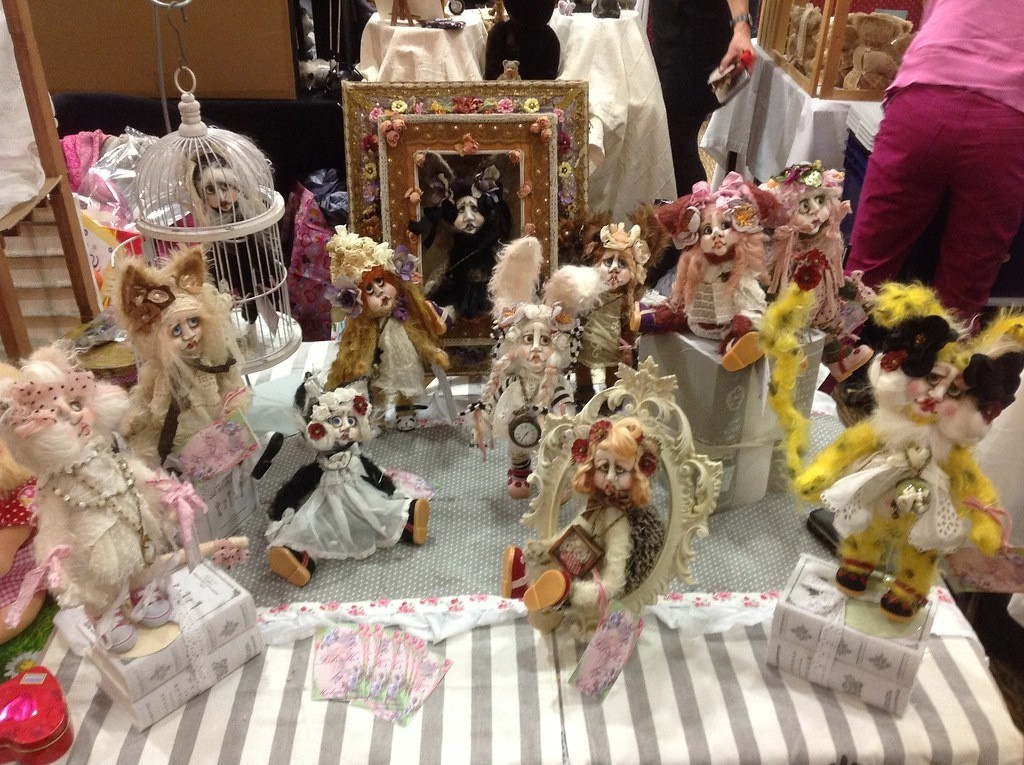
[494,60,522,81]
[788,6,916,91]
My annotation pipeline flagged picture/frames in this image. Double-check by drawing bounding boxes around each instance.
[339,81,590,375]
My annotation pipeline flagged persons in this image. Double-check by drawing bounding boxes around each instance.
[647,0,756,198]
[846,0,1024,332]
[1,153,1024,653]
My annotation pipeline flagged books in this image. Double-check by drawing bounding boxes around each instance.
[77,563,263,733]
[806,508,838,550]
[766,555,937,715]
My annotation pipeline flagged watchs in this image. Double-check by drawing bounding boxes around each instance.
[729,13,753,27]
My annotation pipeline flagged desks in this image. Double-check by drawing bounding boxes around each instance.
[698,18,923,186]
[356,11,677,222]
[0,336,1023,765]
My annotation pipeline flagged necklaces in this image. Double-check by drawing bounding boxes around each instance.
[507,375,541,448]
[373,316,389,376]
[547,509,624,579]
[52,451,156,566]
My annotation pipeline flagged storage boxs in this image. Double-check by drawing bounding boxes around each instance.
[637,321,827,521]
